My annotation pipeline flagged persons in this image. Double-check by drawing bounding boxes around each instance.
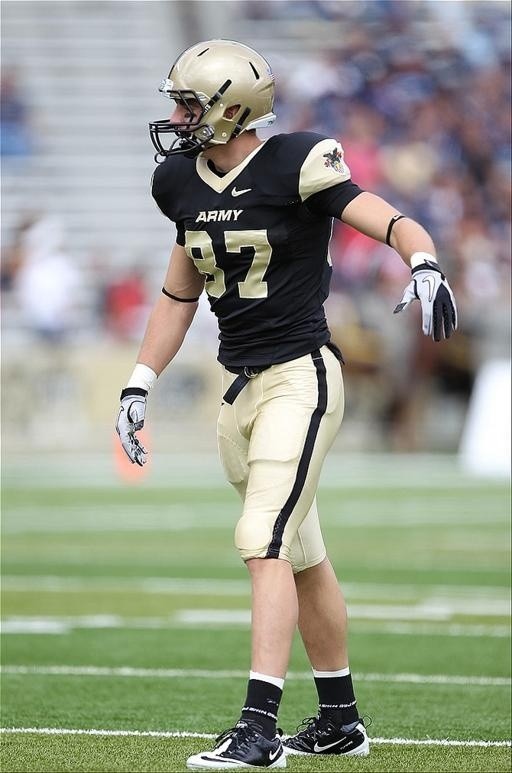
[116,38,459,769]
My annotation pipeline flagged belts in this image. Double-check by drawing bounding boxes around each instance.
[219,362,267,407]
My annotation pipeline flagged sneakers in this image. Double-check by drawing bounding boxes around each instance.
[282,716,373,759]
[187,720,292,773]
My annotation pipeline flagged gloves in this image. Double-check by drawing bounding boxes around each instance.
[117,387,148,468]
[393,260,460,344]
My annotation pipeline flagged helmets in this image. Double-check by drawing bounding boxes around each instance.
[149,37,279,165]
